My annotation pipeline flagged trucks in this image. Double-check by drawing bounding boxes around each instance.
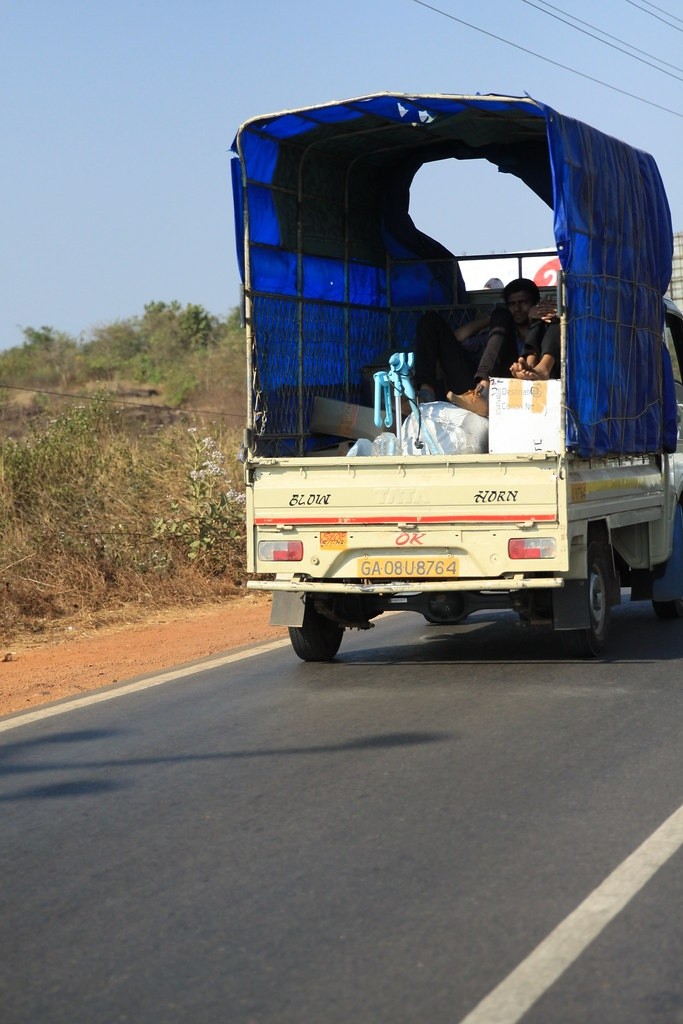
[231,88,683,660]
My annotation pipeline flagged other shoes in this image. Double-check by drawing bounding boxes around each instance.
[392,390,429,416]
[446,384,488,417]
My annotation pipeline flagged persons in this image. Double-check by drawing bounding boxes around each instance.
[392,278,561,416]
[510,313,561,380]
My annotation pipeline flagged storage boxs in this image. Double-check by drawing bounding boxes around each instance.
[491,376,562,453]
